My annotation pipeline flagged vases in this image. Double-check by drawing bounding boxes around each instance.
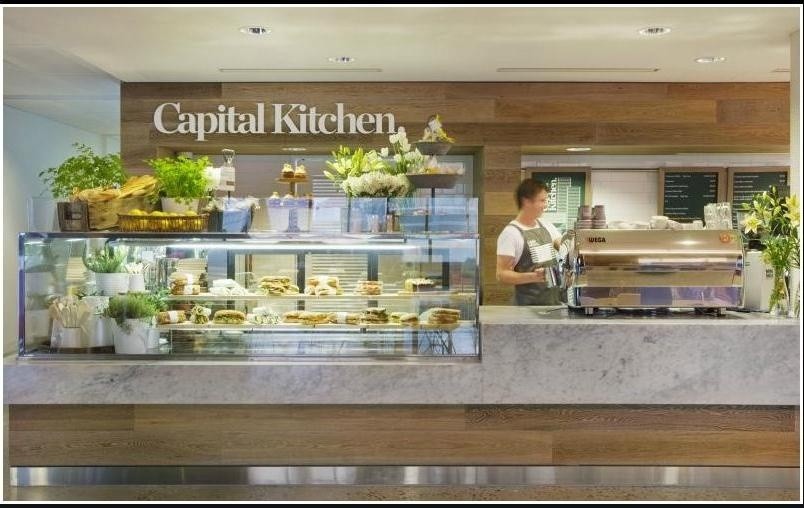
[768,264,800,316]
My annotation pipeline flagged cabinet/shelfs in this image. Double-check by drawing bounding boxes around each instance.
[17,229,482,361]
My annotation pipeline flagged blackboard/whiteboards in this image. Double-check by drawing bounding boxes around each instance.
[658,166,726,227]
[727,166,790,246]
[525,167,592,239]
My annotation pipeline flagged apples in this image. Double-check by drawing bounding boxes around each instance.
[122,209,203,231]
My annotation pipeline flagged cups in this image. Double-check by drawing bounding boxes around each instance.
[578,202,735,230]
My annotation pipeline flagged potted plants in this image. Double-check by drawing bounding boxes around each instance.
[146,150,213,233]
[37,141,126,235]
[69,243,173,354]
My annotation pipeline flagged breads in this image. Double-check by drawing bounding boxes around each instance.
[155,277,460,324]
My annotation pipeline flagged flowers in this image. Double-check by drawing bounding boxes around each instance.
[740,184,800,300]
[322,126,432,231]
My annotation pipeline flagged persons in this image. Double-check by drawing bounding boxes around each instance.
[494,179,570,304]
